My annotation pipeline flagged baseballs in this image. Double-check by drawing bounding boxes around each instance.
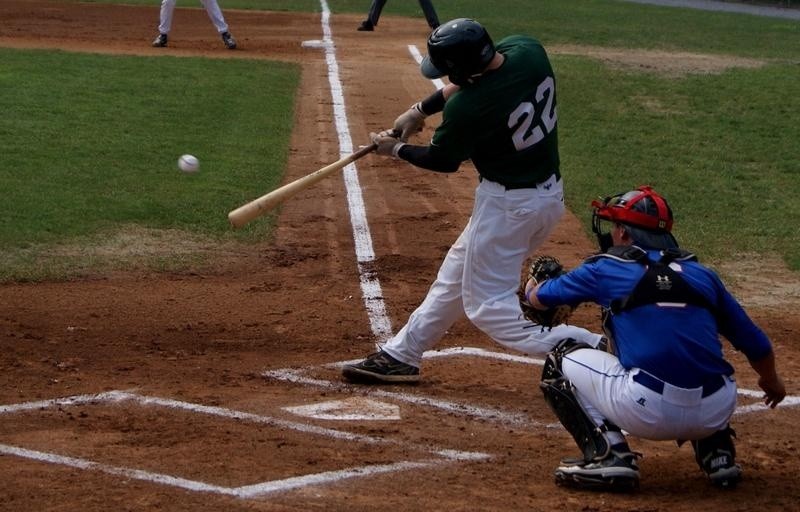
[178,155,200,173]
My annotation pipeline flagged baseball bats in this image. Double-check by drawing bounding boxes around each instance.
[227,123,423,228]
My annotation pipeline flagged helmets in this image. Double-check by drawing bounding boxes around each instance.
[590,185,674,234]
[420,17,497,82]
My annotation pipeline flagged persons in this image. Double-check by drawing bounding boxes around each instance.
[524,185,786,493]
[152,1,237,50]
[340,16,615,384]
[357,0,440,32]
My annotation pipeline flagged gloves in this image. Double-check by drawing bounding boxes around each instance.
[527,253,565,286]
[358,127,410,163]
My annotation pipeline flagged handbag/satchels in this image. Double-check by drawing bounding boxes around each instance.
[393,101,429,143]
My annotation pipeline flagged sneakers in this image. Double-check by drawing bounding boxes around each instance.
[690,422,743,483]
[151,33,167,47]
[341,349,421,386]
[356,21,374,31]
[221,31,236,49]
[554,450,643,494]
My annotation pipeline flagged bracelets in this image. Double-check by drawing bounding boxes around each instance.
[421,88,446,116]
[525,287,535,308]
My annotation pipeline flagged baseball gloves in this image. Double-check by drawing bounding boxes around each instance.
[517,254,581,325]
